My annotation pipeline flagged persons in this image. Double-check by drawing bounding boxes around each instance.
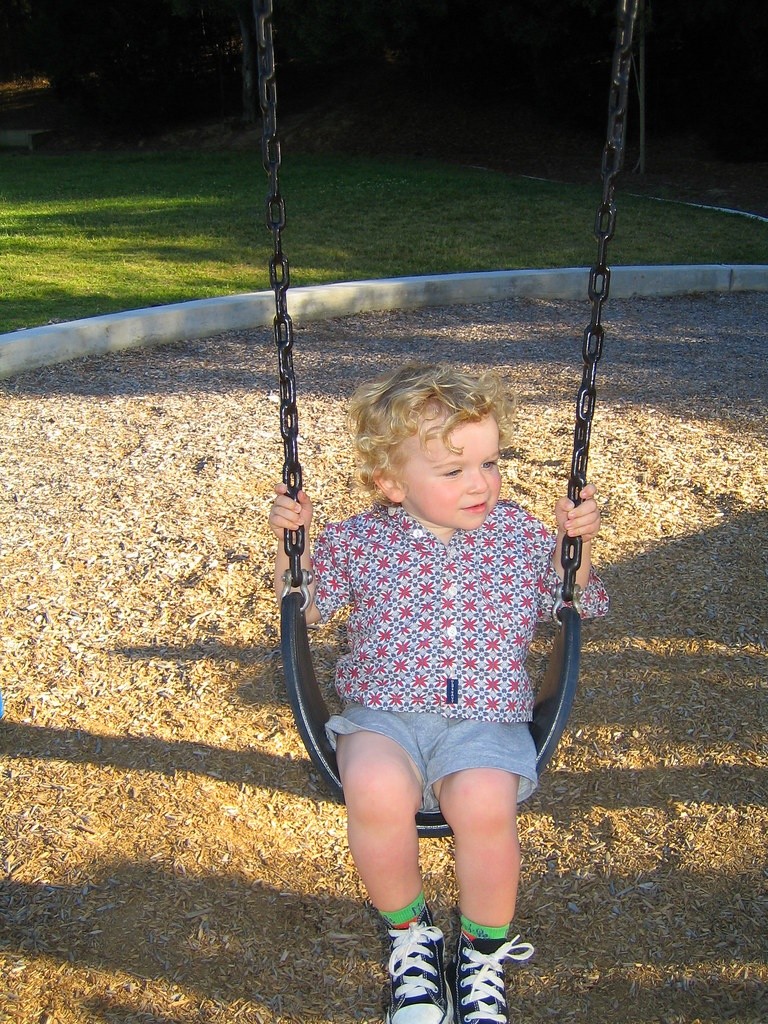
[268,361,609,1024]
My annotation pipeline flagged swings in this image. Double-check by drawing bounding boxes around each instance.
[249,0,646,839]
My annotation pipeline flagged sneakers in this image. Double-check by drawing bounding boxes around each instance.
[382,897,454,1024]
[443,929,535,1024]
